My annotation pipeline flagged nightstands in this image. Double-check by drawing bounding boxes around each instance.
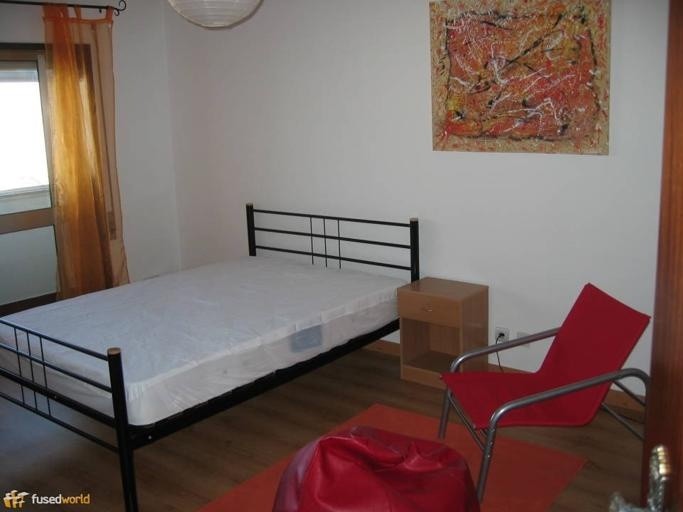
[397,278,489,390]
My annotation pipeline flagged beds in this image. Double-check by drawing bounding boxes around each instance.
[0,204,419,512]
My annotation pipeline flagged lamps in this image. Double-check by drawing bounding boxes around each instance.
[168,0,263,28]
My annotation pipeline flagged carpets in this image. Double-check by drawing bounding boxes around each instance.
[196,404,588,512]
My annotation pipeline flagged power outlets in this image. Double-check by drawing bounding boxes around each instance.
[495,328,509,343]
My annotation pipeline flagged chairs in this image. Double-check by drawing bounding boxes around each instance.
[439,283,650,503]
